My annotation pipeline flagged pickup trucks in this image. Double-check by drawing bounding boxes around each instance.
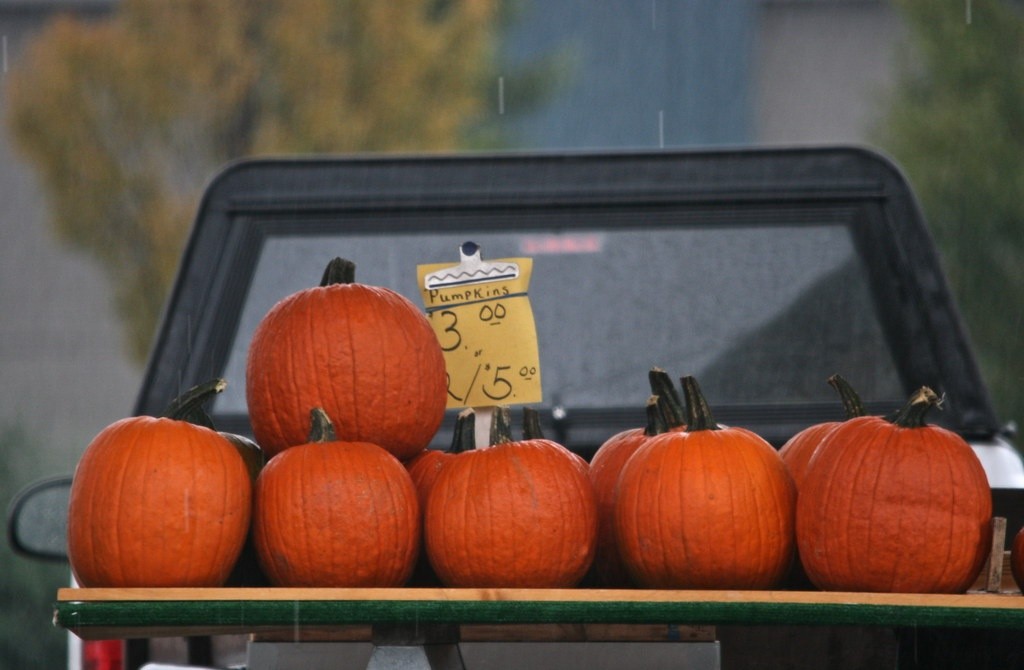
[6,138,1024,670]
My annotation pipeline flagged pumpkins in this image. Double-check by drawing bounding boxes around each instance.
[64,254,1024,595]
